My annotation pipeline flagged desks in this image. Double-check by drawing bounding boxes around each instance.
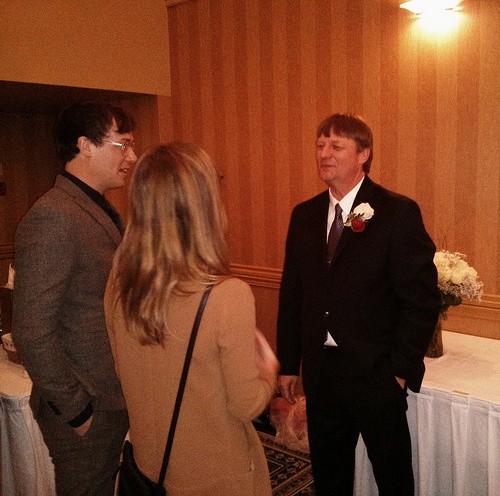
[354,330,500,496]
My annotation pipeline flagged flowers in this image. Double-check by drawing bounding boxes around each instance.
[433,248,484,322]
[343,202,375,232]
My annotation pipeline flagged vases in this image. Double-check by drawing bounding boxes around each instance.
[425,323,444,359]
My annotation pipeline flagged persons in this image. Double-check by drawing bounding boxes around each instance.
[11,102,137,496]
[104,142,281,495]
[275,114,438,496]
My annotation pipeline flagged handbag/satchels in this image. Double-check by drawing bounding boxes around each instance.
[116,440,172,496]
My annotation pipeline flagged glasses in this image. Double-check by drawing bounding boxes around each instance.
[104,138,135,154]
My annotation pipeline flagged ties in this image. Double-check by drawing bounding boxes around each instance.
[327,202,345,262]
